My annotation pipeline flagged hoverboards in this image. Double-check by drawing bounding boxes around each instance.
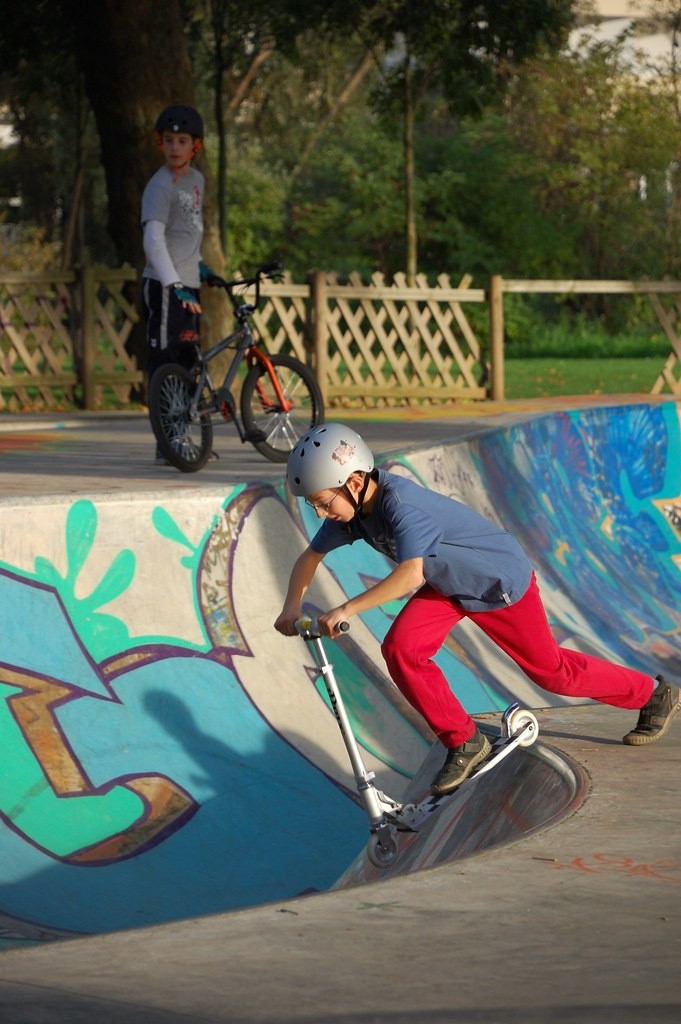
[287,615,542,869]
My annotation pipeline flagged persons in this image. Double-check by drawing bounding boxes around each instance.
[141,106,222,464]
[274,424,680,793]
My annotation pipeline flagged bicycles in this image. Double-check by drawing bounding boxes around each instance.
[147,262,326,473]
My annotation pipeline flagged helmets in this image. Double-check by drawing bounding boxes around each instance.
[287,423,378,497]
[154,105,205,140]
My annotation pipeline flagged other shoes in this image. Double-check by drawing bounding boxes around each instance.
[155,443,218,467]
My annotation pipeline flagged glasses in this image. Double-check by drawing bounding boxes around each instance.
[304,484,345,509]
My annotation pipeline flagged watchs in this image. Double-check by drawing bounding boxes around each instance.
[172,283,183,291]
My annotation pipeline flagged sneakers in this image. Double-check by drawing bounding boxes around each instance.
[429,729,492,794]
[621,675,681,745]
